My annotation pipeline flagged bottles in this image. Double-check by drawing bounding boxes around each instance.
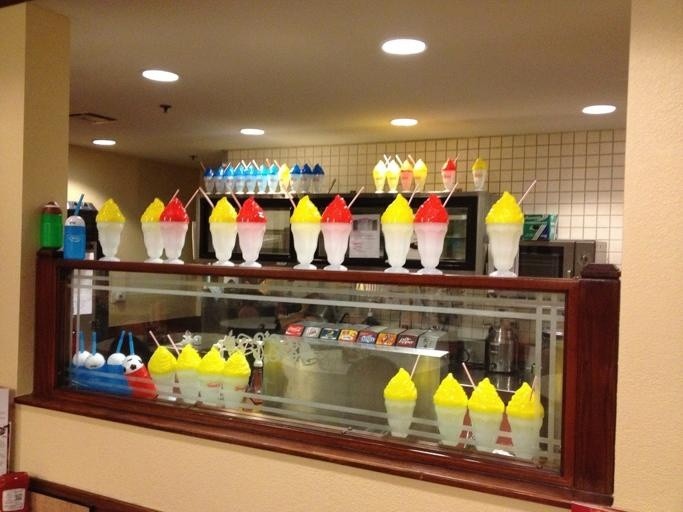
[39,201,87,259]
[72,350,105,391]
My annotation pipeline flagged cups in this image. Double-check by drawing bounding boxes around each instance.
[95,158,544,460]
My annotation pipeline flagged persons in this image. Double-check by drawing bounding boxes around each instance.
[274,292,324,329]
[238,291,265,319]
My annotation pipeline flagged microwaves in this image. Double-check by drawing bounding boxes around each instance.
[519,240,607,278]
